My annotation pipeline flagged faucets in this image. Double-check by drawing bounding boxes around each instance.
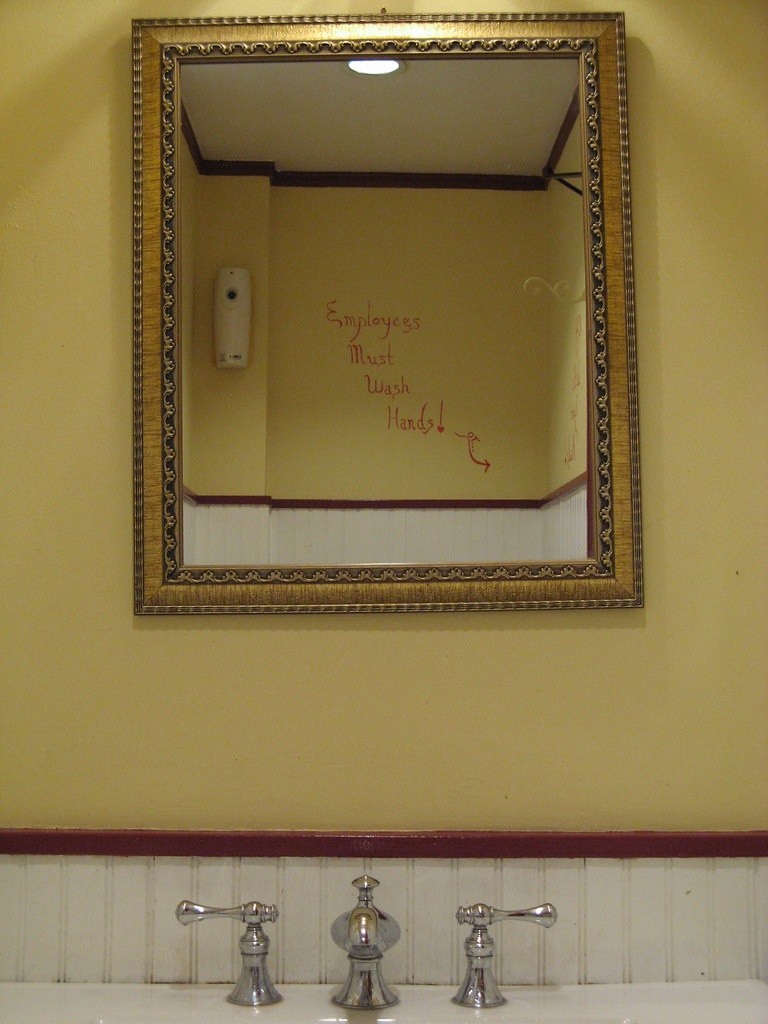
[326,875,401,1012]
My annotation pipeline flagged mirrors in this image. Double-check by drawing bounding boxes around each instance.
[132,12,647,610]
